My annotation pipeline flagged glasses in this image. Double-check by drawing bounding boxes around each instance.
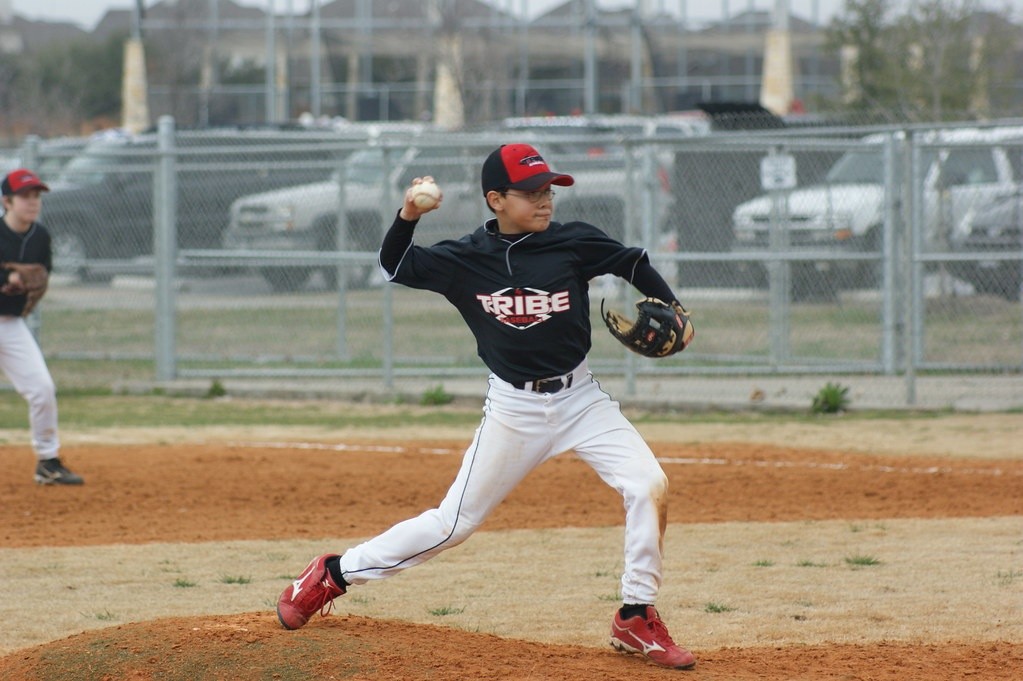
[502,189,555,202]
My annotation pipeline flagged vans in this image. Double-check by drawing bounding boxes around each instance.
[489,111,712,235]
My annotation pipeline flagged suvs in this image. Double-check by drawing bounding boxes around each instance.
[30,118,362,285]
[730,125,1023,304]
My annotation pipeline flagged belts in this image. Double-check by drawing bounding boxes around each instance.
[512,372,574,392]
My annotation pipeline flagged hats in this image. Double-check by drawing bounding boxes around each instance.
[2,170,49,195]
[482,143,574,197]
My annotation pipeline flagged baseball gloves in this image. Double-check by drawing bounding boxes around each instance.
[599,295,693,360]
[2,261,50,317]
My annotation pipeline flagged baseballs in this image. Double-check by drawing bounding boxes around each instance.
[411,181,440,208]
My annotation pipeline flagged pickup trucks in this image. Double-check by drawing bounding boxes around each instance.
[221,135,653,292]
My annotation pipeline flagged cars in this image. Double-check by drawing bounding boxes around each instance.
[944,189,1022,301]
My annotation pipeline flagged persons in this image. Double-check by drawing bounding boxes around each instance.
[277,145,696,670]
[0,169,84,485]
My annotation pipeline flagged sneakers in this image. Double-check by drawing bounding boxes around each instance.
[276,552,347,630]
[608,606,696,670]
[33,459,84,485]
